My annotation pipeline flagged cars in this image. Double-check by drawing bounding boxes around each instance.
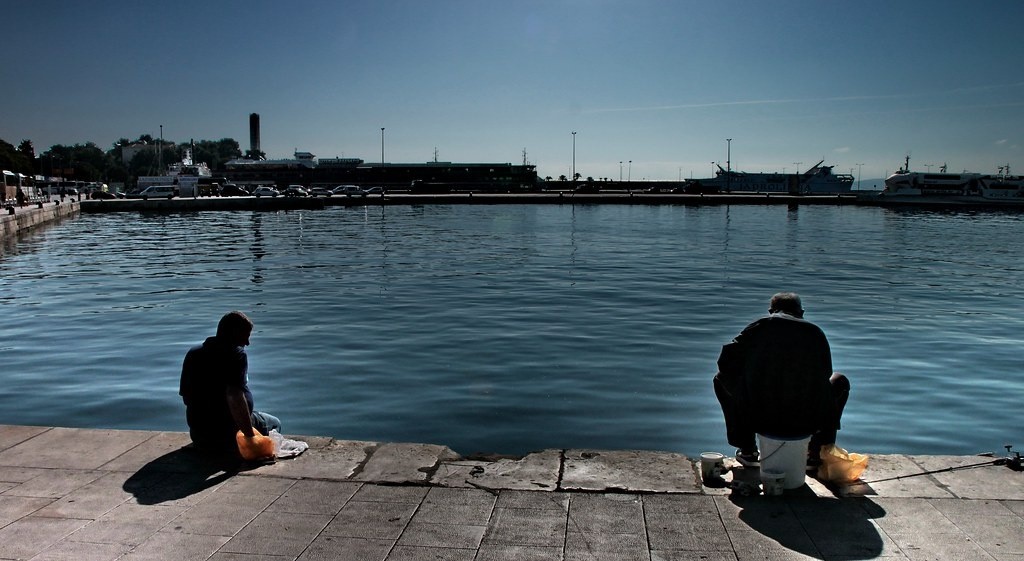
[309,187,334,197]
[91,191,116,199]
[110,182,372,198]
[252,186,281,197]
[366,186,385,195]
[284,188,309,197]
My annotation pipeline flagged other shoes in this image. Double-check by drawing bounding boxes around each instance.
[736,448,761,466]
[806,455,818,469]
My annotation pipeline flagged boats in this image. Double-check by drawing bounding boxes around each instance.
[884,154,1024,199]
[714,158,855,196]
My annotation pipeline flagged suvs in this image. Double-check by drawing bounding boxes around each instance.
[342,186,370,198]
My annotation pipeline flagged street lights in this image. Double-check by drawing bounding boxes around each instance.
[856,164,865,191]
[572,131,577,195]
[381,127,385,187]
[628,161,632,182]
[726,138,732,193]
[711,161,714,179]
[620,162,623,182]
[160,125,163,175]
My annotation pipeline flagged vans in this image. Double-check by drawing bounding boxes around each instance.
[139,185,175,200]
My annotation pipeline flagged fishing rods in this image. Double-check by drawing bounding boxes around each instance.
[847,443,1024,488]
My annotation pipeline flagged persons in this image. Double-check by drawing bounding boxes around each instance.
[179,312,282,457]
[713,292,851,471]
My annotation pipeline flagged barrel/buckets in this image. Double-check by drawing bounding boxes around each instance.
[700,452,724,479]
[757,434,811,489]
[762,469,785,496]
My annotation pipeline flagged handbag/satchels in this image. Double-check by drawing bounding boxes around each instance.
[237,427,273,460]
[816,443,868,483]
